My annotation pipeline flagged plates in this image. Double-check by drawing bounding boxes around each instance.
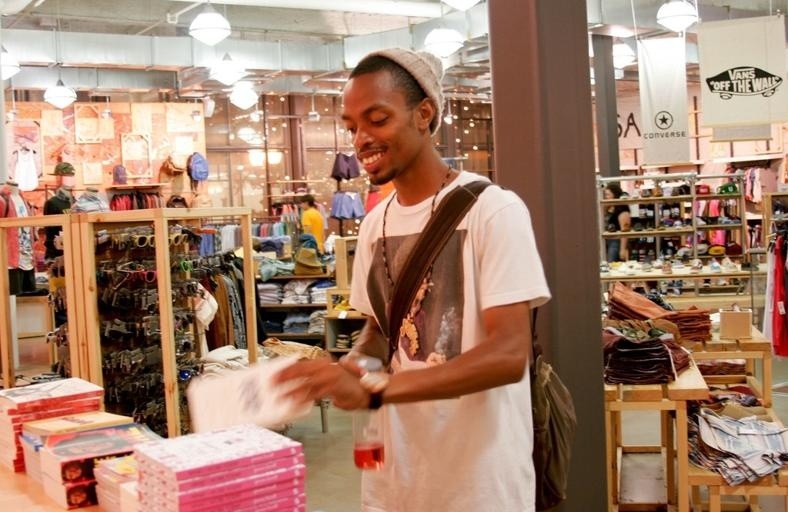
[599,320,788,512]
[597,170,751,294]
[323,238,366,360]
[10,292,59,372]
[761,193,787,253]
[251,273,339,351]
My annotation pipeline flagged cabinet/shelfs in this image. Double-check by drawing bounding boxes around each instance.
[354,359,384,470]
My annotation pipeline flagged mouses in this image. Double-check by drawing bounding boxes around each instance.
[205,249,236,280]
[766,223,787,253]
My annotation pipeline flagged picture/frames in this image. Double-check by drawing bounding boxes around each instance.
[0,466,103,511]
[602,263,766,327]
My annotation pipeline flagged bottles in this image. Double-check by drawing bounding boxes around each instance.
[48,163,75,175]
[360,48,445,138]
[297,247,322,267]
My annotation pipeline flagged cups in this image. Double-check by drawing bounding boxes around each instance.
[132,229,203,380]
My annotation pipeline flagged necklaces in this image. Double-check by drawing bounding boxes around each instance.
[381,164,453,359]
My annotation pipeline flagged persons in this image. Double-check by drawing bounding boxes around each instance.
[605,184,632,263]
[268,46,553,512]
[299,195,326,258]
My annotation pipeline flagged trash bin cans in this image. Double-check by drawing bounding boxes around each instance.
[360,371,391,411]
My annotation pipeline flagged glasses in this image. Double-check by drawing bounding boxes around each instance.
[0,2,704,173]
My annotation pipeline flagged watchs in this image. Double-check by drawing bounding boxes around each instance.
[360,371,391,411]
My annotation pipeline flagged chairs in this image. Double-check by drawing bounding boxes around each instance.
[620,183,737,199]
[600,258,739,274]
[607,215,741,232]
[636,278,744,294]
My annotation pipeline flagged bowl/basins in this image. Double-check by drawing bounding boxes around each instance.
[381,164,453,359]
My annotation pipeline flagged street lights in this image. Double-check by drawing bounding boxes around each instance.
[191,154,208,180]
[530,355,577,512]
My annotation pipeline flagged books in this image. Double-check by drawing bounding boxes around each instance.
[134,422,305,510]
[2,377,107,472]
[19,410,165,511]
